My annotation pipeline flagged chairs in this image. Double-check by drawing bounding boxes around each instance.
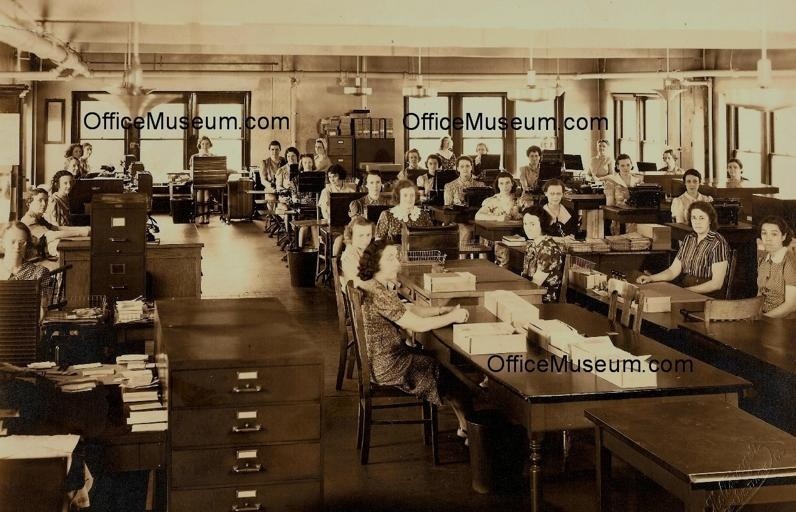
[123,155,144,183]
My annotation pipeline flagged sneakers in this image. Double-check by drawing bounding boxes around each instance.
[198,215,209,224]
[457,425,469,445]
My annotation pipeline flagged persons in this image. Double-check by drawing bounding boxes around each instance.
[188,134,216,226]
[0,141,95,315]
[258,134,796,447]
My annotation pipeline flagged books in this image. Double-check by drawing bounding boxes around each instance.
[28,351,170,432]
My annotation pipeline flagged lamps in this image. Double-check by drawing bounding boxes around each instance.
[87,22,183,124]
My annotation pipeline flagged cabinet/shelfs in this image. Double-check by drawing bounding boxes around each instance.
[326,136,395,182]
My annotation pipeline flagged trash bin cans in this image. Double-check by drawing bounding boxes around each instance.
[286,247,319,288]
[169,197,195,224]
[464,407,528,496]
